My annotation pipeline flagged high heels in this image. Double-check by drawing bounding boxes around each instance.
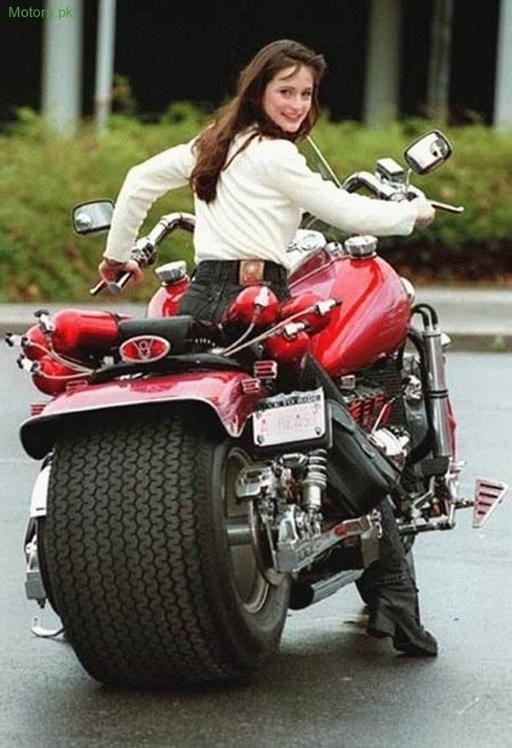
[366,579,440,657]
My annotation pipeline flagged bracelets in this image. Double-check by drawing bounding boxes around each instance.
[105,257,123,268]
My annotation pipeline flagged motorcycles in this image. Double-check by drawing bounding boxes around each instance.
[4,128,510,690]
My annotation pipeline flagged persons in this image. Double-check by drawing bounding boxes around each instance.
[97,35,442,662]
